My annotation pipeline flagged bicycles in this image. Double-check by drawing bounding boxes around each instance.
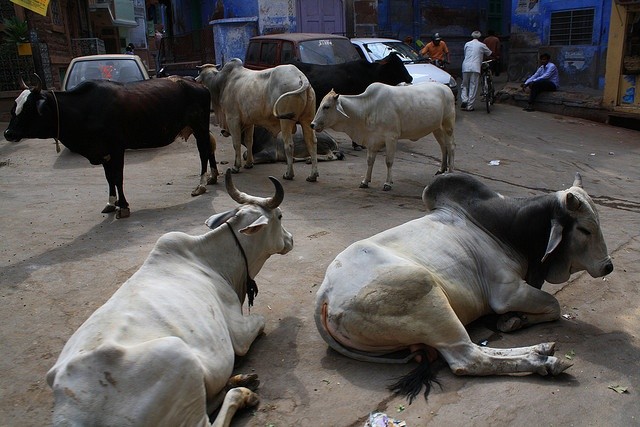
[481,60,496,112]
[429,59,446,69]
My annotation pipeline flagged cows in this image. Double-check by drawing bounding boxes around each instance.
[221,124,347,165]
[3,72,221,220]
[284,51,414,152]
[45,168,295,427]
[310,81,458,193]
[195,58,320,183]
[314,172,617,407]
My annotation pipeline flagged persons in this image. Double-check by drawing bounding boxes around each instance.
[521,53,561,110]
[461,30,494,111]
[420,32,452,68]
[484,30,501,76]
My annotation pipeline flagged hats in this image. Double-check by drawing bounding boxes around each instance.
[432,33,442,40]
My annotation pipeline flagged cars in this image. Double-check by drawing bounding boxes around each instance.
[59,54,149,92]
[330,31,461,106]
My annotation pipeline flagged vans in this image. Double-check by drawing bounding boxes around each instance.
[244,33,360,69]
